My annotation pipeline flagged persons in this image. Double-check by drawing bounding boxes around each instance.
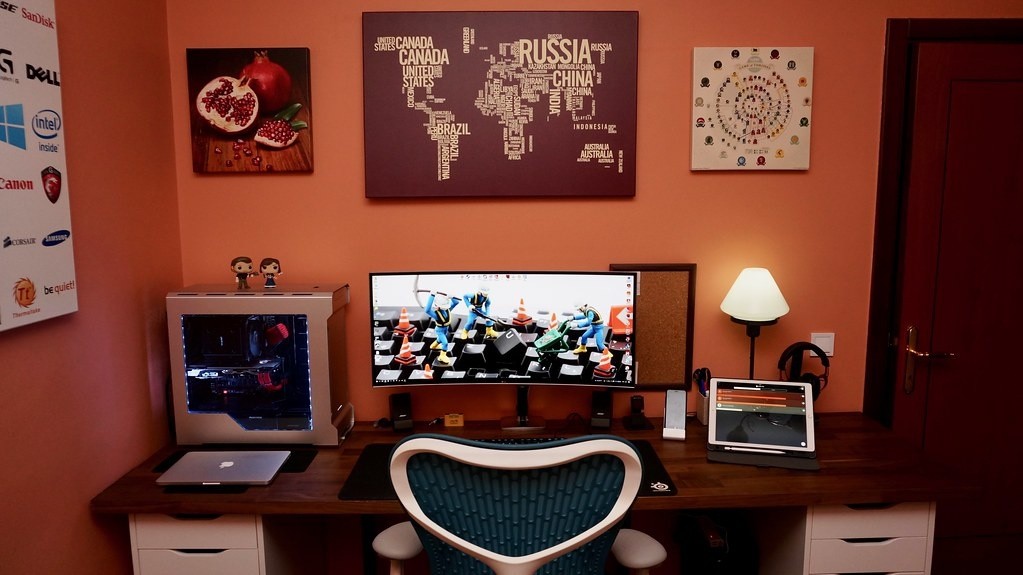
[461,289,499,340]
[567,303,608,355]
[231,257,259,290]
[424,288,460,364]
[260,258,283,288]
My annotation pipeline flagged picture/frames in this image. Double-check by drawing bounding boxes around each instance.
[691,45,814,172]
[609,261,697,395]
[362,8,640,201]
[184,44,315,176]
[0,0,80,341]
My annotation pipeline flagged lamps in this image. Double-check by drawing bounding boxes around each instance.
[720,267,791,381]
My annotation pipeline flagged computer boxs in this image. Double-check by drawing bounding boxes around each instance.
[166,283,355,446]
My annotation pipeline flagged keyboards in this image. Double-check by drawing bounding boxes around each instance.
[471,438,570,445]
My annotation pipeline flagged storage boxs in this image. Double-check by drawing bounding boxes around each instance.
[165,276,358,449]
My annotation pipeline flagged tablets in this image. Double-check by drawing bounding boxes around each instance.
[708,378,815,454]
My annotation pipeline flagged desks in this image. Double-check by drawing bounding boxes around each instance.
[84,410,974,575]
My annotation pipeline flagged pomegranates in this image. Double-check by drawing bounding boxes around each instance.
[197,51,307,172]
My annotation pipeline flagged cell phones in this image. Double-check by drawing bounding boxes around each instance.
[663,390,685,432]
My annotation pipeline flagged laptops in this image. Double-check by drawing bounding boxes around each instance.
[155,451,292,486]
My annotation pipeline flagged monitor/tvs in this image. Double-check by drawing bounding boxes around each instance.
[368,271,640,388]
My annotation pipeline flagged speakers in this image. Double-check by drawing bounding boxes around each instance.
[390,391,414,435]
[590,391,613,429]
